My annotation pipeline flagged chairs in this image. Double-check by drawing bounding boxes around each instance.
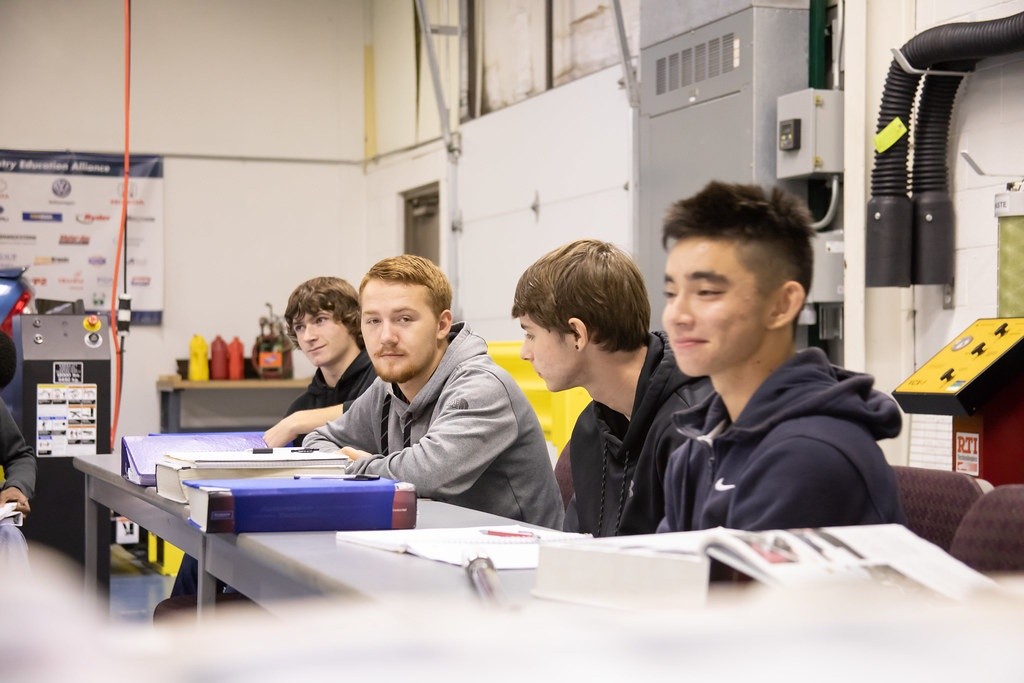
[885,464,993,550]
[951,483,1024,573]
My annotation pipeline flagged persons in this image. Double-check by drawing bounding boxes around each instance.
[0,253,566,628]
[512,241,711,538]
[655,178,903,533]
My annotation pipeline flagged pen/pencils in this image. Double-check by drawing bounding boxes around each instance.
[478,528,542,539]
[249,446,319,453]
[294,473,382,481]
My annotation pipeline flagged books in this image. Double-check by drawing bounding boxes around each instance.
[121,432,991,616]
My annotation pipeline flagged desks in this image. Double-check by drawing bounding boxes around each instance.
[158,378,314,432]
[70,452,593,669]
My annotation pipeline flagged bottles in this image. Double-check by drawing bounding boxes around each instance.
[212,336,229,380]
[229,337,244,380]
[188,334,209,381]
